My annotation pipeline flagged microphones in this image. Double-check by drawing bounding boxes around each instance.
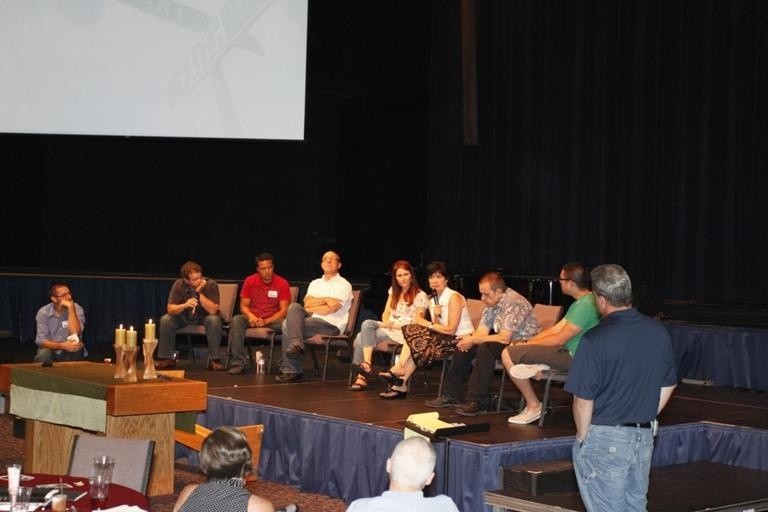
[431,289,441,318]
[191,292,199,317]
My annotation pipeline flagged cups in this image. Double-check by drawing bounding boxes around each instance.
[7,486,32,512]
[88,454,115,510]
[51,494,67,512]
[5,464,23,503]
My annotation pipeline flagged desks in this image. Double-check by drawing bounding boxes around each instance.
[0,467,150,511]
[3,358,212,502]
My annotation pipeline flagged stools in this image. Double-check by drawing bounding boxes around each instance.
[516,364,571,428]
[348,334,432,394]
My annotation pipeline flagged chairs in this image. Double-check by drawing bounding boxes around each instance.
[176,283,239,370]
[305,290,362,383]
[468,303,562,416]
[66,434,156,497]
[402,300,487,399]
[225,286,299,375]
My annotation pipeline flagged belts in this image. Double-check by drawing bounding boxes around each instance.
[604,422,652,428]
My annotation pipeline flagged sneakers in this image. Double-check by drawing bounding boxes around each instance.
[508,402,546,424]
[511,364,551,379]
[286,345,305,360]
[275,373,304,382]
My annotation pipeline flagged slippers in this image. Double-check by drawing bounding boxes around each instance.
[379,389,407,400]
[378,372,404,386]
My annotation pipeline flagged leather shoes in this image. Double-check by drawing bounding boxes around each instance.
[425,395,462,408]
[455,401,487,416]
[229,365,244,374]
[210,359,225,370]
[155,360,177,369]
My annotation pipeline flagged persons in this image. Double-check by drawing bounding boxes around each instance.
[228,252,292,374]
[33,283,88,362]
[154,262,228,371]
[345,436,460,512]
[173,426,274,512]
[275,251,354,383]
[562,265,679,512]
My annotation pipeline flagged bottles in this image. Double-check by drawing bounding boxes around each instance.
[258,354,265,376]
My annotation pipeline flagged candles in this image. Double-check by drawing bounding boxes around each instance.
[115,328,126,346]
[126,330,137,348]
[145,323,156,339]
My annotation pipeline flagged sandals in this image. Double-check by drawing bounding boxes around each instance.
[351,362,374,375]
[349,374,368,391]
